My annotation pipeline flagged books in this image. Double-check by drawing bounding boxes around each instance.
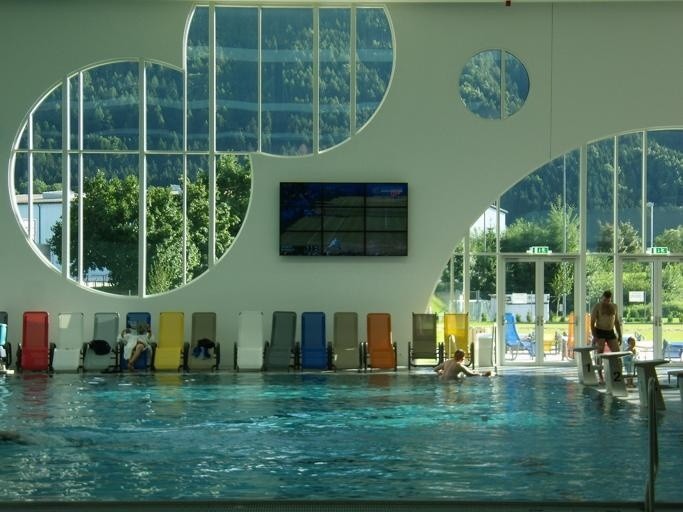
[128,320,146,335]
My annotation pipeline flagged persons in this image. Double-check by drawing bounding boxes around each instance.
[120,319,152,372]
[591,291,622,385]
[434,349,491,382]
[623,337,637,388]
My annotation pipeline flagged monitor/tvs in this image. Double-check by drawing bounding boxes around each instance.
[279,181,409,256]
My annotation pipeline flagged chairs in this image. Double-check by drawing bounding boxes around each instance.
[363,312,398,372]
[494,312,546,359]
[83,314,118,373]
[441,312,475,371]
[50,313,83,373]
[234,312,263,371]
[186,313,220,371]
[330,312,363,371]
[152,312,186,373]
[1,312,12,370]
[263,312,297,371]
[556,311,593,360]
[297,312,330,372]
[118,312,151,373]
[408,312,441,372]
[16,312,50,373]
